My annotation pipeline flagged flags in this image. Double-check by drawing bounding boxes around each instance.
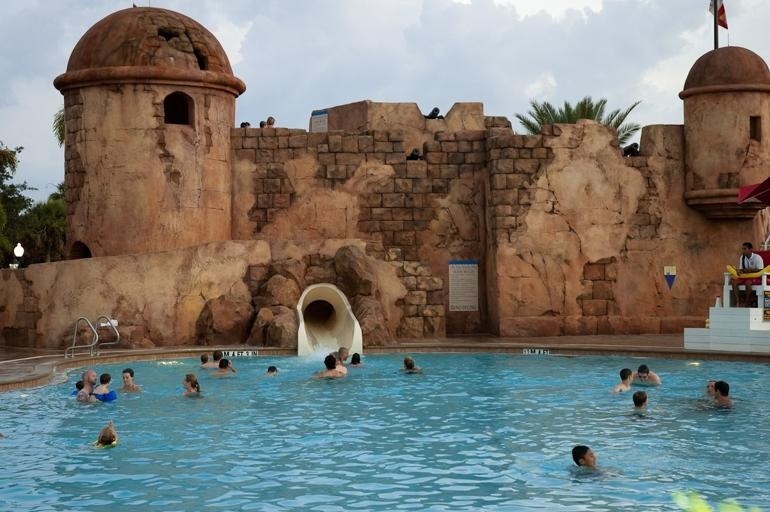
[708,0,729,29]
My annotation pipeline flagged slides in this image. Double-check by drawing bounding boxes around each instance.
[296,282,364,361]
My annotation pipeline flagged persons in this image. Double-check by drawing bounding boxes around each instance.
[401,356,420,371]
[314,346,360,378]
[201,350,237,375]
[614,368,633,393]
[268,366,277,376]
[732,243,764,307]
[182,373,200,395]
[714,381,730,408]
[72,368,141,403]
[633,391,648,409]
[631,364,661,385]
[241,117,274,128]
[572,445,596,467]
[707,381,717,396]
[92,425,116,448]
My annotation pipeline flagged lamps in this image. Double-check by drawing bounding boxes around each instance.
[12,242,25,268]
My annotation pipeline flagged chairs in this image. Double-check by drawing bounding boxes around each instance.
[723,250,770,307]
